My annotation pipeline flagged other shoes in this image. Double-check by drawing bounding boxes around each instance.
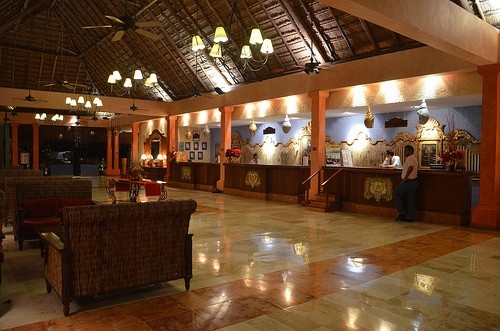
[395,213,413,222]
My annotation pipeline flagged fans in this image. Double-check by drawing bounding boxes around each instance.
[37,74,88,90]
[81,15,162,41]
[121,104,148,112]
[184,87,213,98]
[12,90,48,103]
[292,58,336,76]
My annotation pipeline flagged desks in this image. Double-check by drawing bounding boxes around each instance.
[105,178,168,203]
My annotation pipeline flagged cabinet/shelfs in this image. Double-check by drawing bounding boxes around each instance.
[138,167,166,182]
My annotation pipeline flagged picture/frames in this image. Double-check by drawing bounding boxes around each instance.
[194,142,199,150]
[419,141,439,168]
[193,130,200,141]
[202,142,207,150]
[185,142,190,150]
[190,152,195,159]
[198,152,203,160]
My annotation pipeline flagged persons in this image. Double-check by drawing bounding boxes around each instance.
[250,153,261,164]
[393,144,421,221]
[378,149,403,169]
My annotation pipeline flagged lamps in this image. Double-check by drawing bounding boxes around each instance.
[364,106,374,129]
[283,114,291,133]
[107,0,158,97]
[417,100,430,125]
[34,113,47,126]
[65,77,104,117]
[191,0,274,72]
[141,154,147,167]
[8,105,18,116]
[51,114,64,125]
[157,153,163,167]
[247,119,258,136]
[214,87,223,95]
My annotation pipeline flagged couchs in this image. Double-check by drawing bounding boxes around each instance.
[13,179,93,250]
[41,199,197,316]
[0,171,82,264]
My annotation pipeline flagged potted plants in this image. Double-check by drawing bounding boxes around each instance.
[98,158,106,176]
